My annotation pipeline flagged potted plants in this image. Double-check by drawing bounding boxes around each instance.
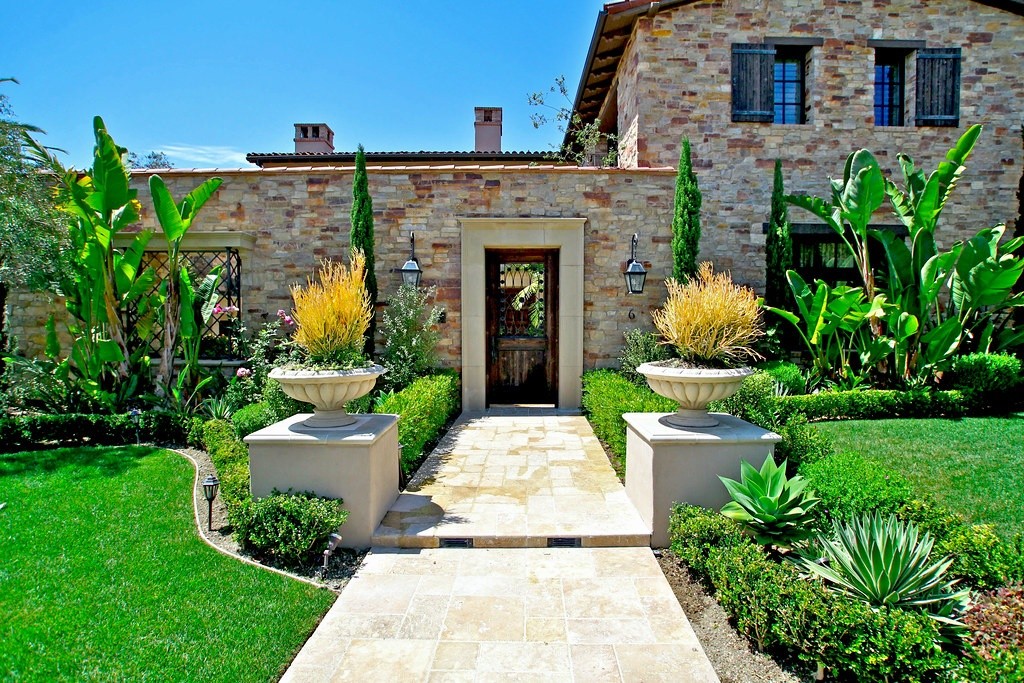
[267,245,388,428]
[636,260,766,427]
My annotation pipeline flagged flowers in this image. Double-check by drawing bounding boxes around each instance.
[213,304,295,379]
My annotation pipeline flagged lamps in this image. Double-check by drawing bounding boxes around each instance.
[389,232,423,290]
[202,473,221,531]
[130,408,141,445]
[623,233,649,295]
